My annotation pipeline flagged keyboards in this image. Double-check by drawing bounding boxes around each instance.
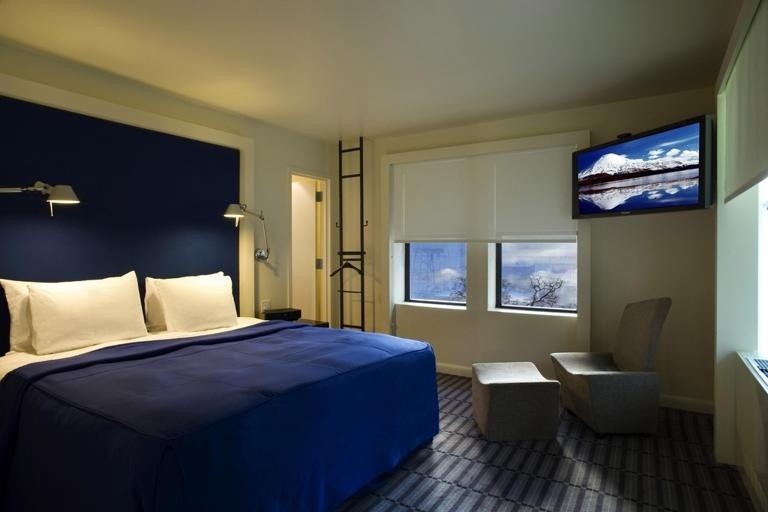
[747,357,768,387]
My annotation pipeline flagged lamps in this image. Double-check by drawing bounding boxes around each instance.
[223,204,270,264]
[0,181,81,216]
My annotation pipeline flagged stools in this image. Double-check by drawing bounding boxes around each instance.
[472,362,561,442]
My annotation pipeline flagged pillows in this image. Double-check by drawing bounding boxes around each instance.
[0,276,119,355]
[27,270,148,355]
[144,271,224,331]
[154,275,237,332]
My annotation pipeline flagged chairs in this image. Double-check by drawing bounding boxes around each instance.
[549,297,672,438]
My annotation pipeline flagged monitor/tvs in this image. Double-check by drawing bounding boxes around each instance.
[572,113,711,219]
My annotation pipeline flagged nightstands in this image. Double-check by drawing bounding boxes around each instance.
[295,319,329,328]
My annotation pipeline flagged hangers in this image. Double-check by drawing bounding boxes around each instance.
[330,259,364,277]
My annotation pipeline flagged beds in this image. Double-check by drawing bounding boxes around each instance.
[0,316,439,512]
